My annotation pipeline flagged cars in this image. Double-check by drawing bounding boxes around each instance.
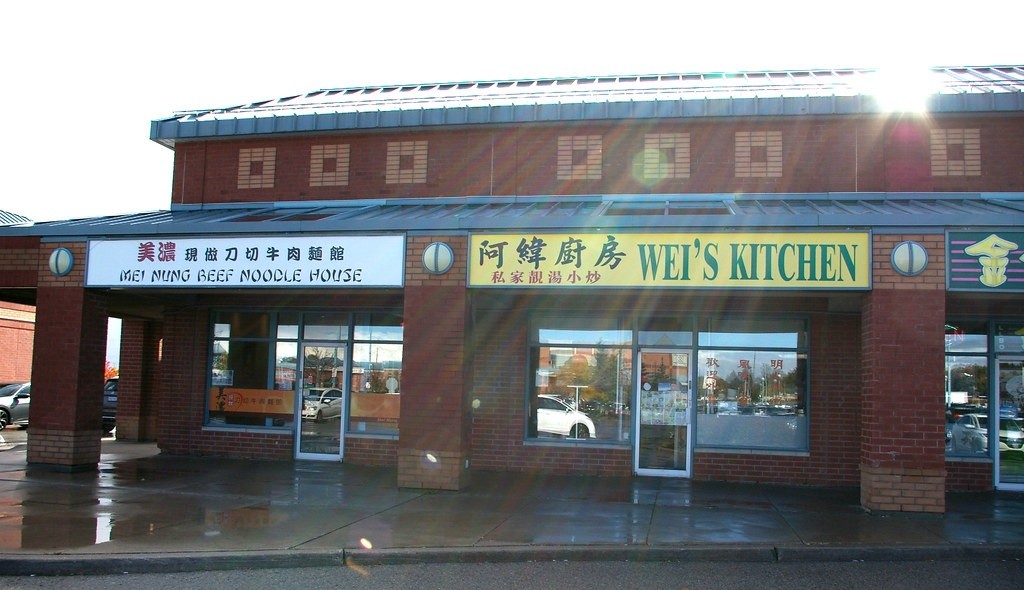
[536,395,596,440]
[946,405,1024,454]
[301,388,343,422]
[102,376,120,427]
[0,382,31,429]
